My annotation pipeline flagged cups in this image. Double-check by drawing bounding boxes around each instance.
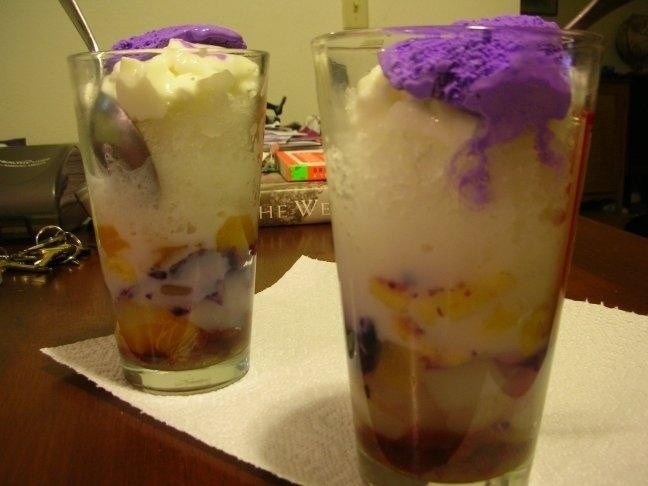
[66,49,272,395]
[311,28,605,485]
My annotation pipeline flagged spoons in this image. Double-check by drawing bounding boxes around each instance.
[55,0,161,203]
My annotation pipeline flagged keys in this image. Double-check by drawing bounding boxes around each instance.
[0,224,90,276]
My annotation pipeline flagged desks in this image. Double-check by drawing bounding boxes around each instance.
[2,210,647,486]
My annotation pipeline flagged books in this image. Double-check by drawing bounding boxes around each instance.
[270,142,328,182]
[255,171,330,228]
[260,128,322,152]
